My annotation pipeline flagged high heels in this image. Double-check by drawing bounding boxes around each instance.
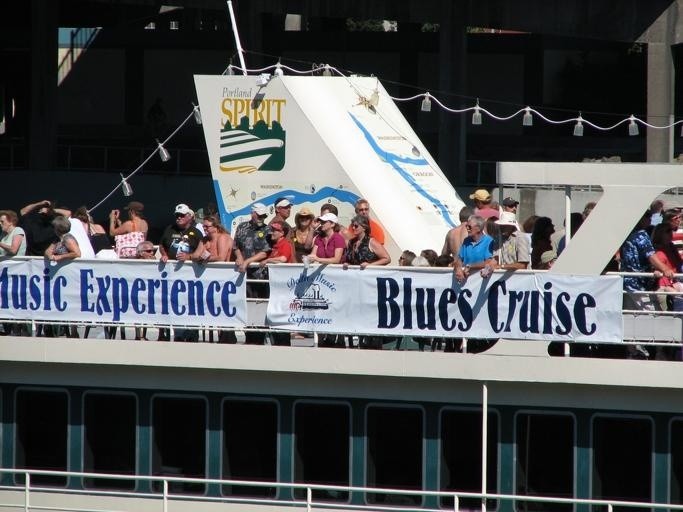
[636,341,650,357]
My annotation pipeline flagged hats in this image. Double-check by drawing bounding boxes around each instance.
[189,209,195,220]
[175,204,189,214]
[317,213,338,225]
[503,197,519,206]
[274,198,293,207]
[251,203,269,216]
[494,212,516,225]
[470,189,491,202]
[297,207,312,215]
[123,202,144,211]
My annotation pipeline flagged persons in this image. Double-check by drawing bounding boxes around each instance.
[470,190,500,220]
[267,197,294,241]
[453,215,495,285]
[303,213,347,347]
[440,205,480,259]
[486,216,500,234]
[1,210,26,336]
[650,200,663,241]
[260,221,296,345]
[343,215,391,348]
[502,197,520,227]
[622,209,673,361]
[558,213,585,257]
[42,208,94,260]
[673,207,683,263]
[480,212,530,278]
[85,212,121,260]
[191,215,236,342]
[45,217,80,336]
[348,199,384,245]
[304,204,352,249]
[649,223,683,359]
[136,241,156,340]
[110,202,148,339]
[583,203,597,217]
[532,217,556,270]
[398,249,453,267]
[233,203,274,344]
[292,207,315,263]
[159,204,203,341]
[19,201,68,256]
[663,209,681,231]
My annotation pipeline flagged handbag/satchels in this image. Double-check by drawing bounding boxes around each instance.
[115,220,146,258]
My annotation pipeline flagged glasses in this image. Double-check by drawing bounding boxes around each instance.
[357,208,368,211]
[176,214,186,218]
[202,226,212,228]
[671,216,680,220]
[351,223,360,228]
[256,214,268,218]
[466,225,477,228]
[269,228,283,232]
[138,250,156,253]
[505,204,517,208]
[400,257,406,260]
